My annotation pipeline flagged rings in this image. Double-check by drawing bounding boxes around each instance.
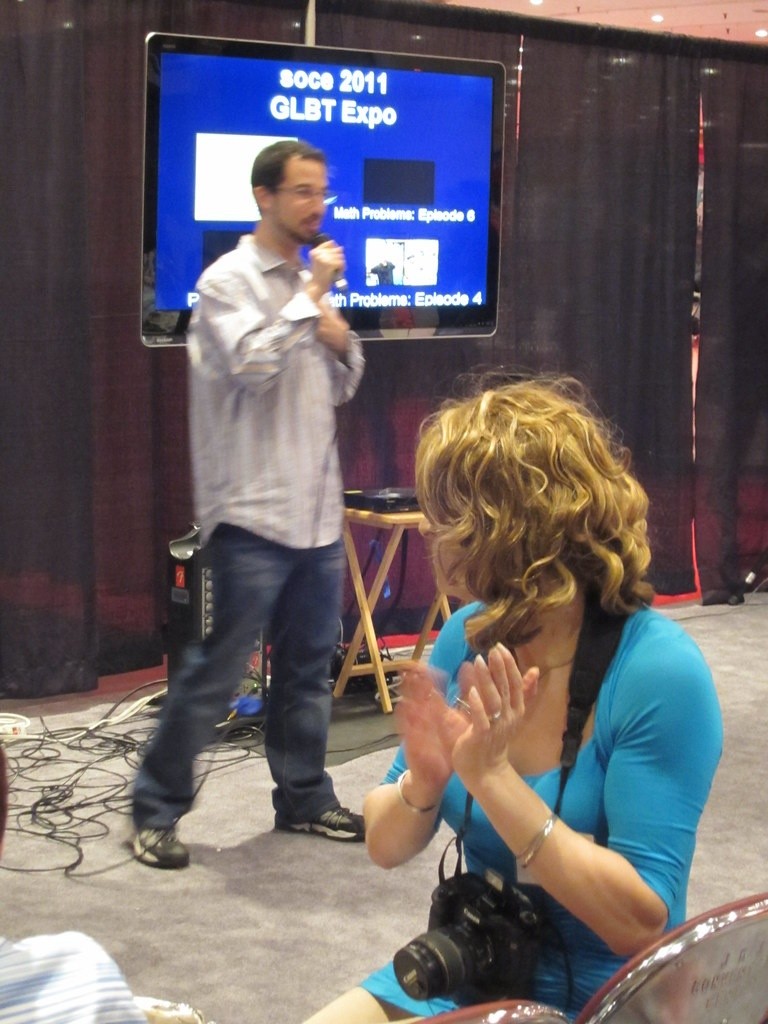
[488,711,502,723]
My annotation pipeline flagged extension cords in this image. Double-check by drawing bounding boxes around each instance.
[0,722,28,736]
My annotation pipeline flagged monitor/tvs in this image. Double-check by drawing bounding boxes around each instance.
[137,30,507,348]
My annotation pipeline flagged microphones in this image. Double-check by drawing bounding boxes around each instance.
[310,233,348,292]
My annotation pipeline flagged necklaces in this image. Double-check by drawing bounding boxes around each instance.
[536,660,576,680]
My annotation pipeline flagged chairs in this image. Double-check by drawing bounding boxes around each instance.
[575,892,767,1024]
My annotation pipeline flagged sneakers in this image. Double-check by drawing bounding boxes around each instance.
[123,824,192,872]
[274,798,363,844]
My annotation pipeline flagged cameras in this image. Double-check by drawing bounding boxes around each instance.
[393,871,550,1007]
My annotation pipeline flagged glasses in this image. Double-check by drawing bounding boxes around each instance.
[272,184,339,207]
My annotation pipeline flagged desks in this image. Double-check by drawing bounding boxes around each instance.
[338,504,458,712]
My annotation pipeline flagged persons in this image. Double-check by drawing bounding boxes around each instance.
[0,744,151,1024]
[115,140,374,870]
[297,363,727,1024]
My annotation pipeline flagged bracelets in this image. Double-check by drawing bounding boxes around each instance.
[517,815,556,867]
[396,768,434,816]
